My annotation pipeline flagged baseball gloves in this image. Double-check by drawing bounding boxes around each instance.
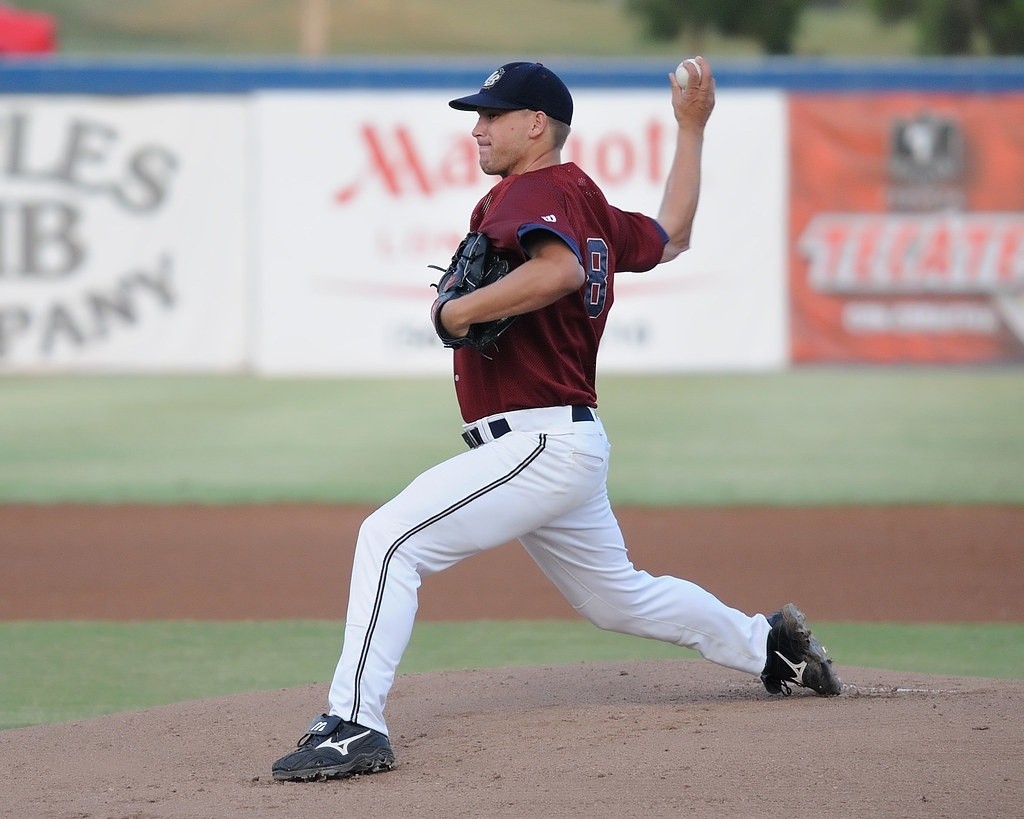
[428,229,525,352]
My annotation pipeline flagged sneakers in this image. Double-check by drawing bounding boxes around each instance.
[272,712,396,779]
[760,602,841,697]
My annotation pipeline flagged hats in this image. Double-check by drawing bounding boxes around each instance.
[449,61,573,126]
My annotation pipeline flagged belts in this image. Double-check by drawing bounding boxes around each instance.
[461,404,594,448]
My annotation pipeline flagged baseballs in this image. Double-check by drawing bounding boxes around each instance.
[675,55,701,88]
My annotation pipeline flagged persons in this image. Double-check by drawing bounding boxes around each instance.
[271,55,841,780]
